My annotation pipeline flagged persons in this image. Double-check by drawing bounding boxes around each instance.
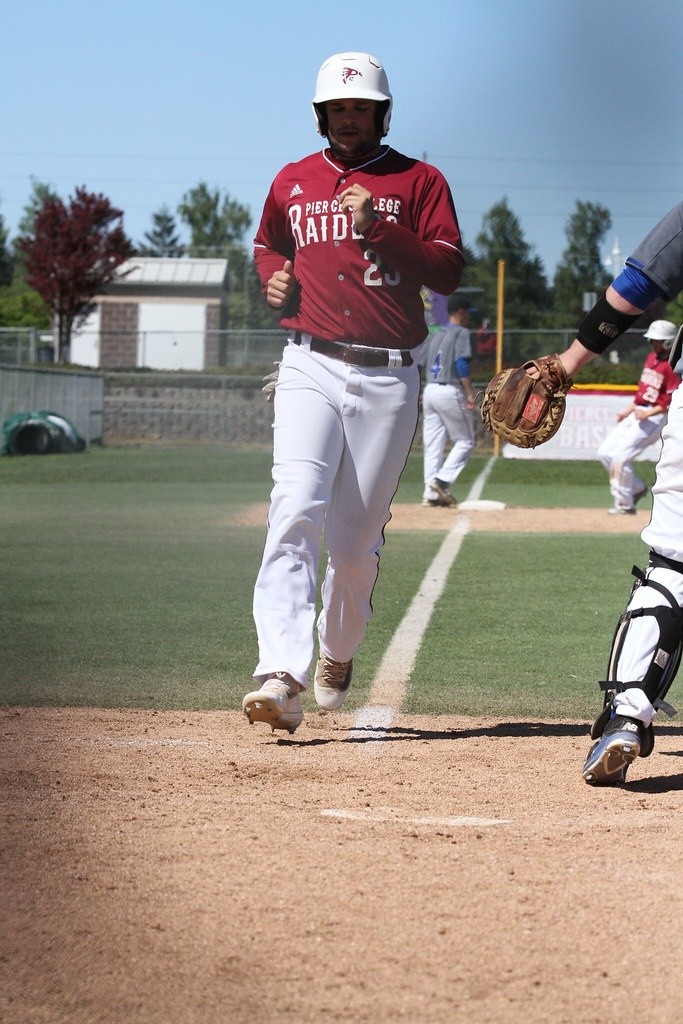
[474,317,497,373]
[481,201,683,787]
[240,53,464,733]
[596,319,677,515]
[418,298,480,507]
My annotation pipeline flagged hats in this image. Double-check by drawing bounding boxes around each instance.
[447,297,479,313]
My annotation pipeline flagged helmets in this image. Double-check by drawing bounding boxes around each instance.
[643,319,676,350]
[311,52,393,138]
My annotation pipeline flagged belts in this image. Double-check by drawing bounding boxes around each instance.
[293,332,414,366]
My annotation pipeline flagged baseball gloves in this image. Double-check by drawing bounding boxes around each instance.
[470,352,578,451]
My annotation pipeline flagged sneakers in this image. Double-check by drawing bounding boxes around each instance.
[582,714,643,787]
[429,478,457,504]
[242,672,304,735]
[608,507,636,515]
[421,498,450,508]
[314,651,353,711]
[633,487,649,506]
